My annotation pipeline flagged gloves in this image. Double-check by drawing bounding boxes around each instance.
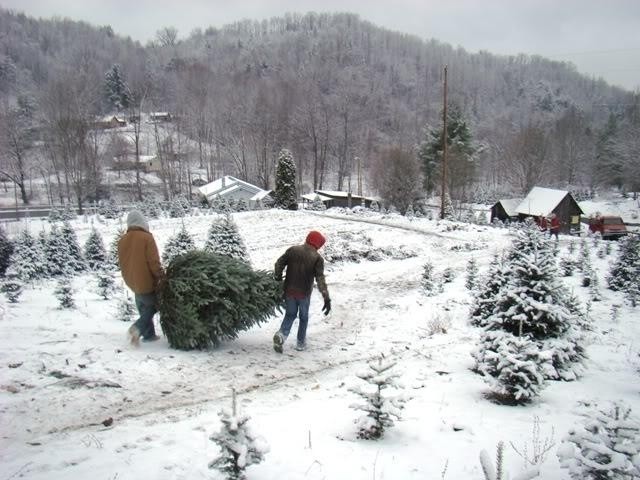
[322,299,331,316]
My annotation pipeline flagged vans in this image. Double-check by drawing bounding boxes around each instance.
[590,215,628,241]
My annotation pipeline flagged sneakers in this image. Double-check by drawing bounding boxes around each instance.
[141,334,161,345]
[297,341,303,351]
[273,331,288,353]
[129,325,140,347]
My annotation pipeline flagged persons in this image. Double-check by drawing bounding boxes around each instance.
[274,230,331,354]
[118,210,165,347]
[537,213,560,242]
